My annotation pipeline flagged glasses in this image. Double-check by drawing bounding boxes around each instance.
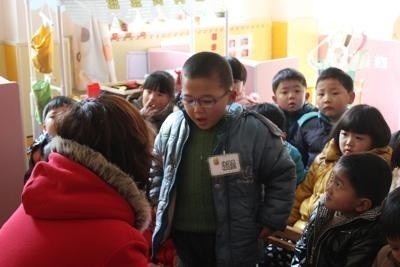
[179,90,229,108]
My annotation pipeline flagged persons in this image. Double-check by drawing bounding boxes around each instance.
[0,52,400,267]
